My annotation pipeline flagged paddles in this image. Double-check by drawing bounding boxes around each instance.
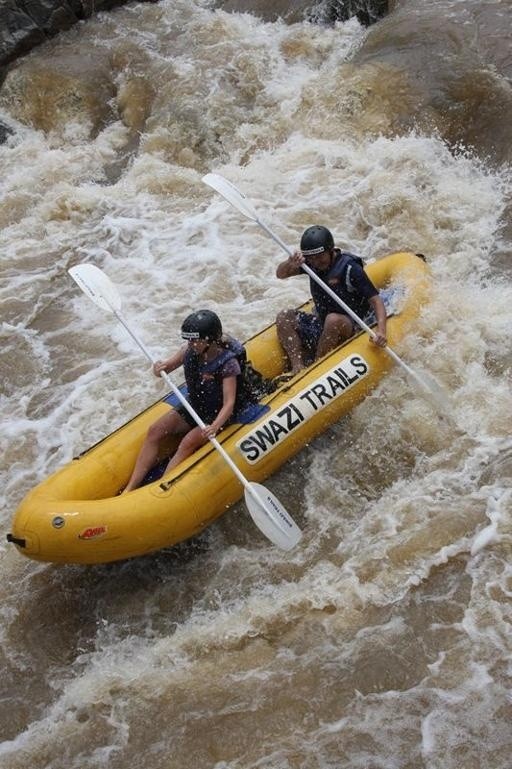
[66,263,303,551]
[201,172,448,409]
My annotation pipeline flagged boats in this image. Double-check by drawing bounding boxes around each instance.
[5,252,430,566]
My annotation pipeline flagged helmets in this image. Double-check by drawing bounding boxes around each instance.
[300,226,334,257]
[181,310,222,341]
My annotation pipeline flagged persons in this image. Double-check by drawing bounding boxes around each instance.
[118,307,248,494]
[269,225,390,393]
